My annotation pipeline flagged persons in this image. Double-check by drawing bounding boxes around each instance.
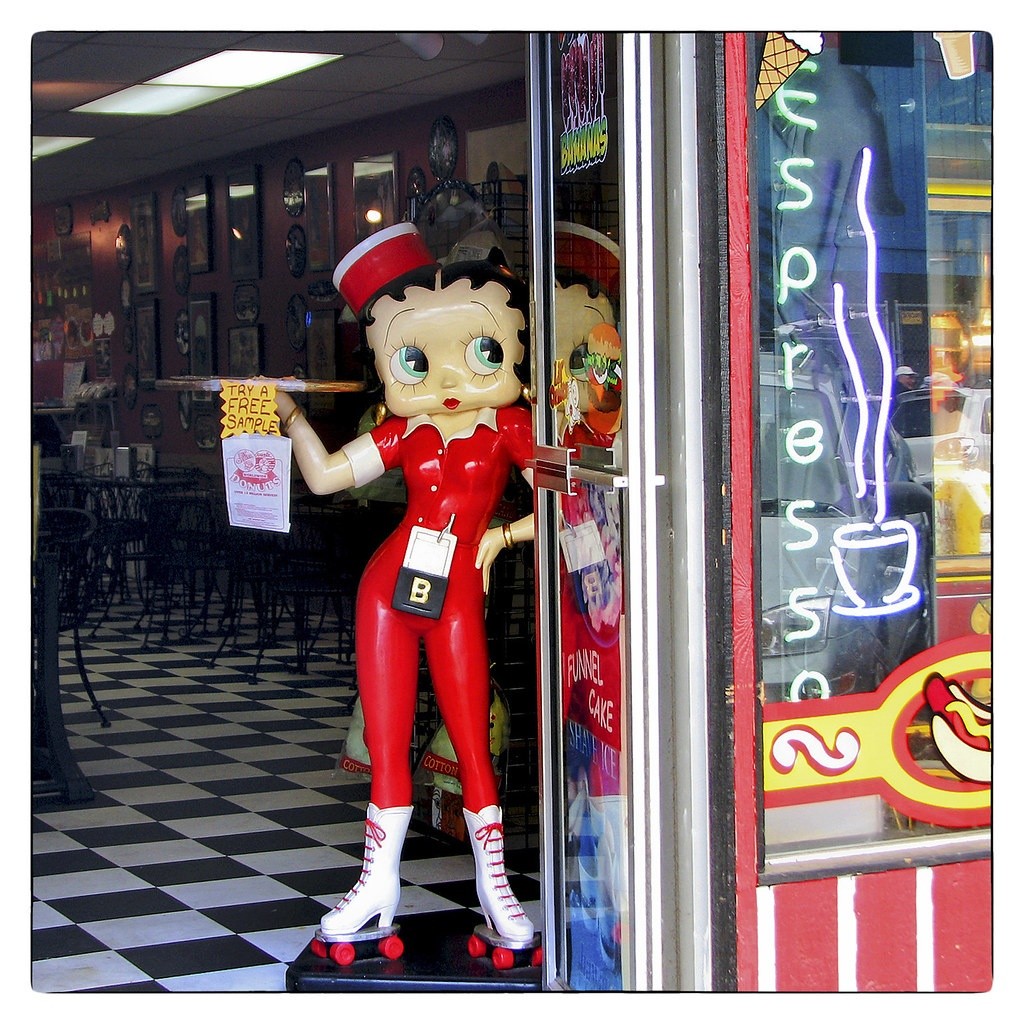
[919,352,961,389]
[885,365,918,419]
[541,218,624,954]
[273,224,540,970]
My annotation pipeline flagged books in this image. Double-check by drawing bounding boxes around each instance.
[59,431,156,480]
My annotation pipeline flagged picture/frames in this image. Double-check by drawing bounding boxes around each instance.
[118,326,134,354]
[117,273,133,322]
[54,204,74,236]
[282,155,304,218]
[285,224,306,279]
[193,417,222,452]
[307,279,338,302]
[427,112,458,179]
[114,224,132,270]
[187,171,214,273]
[141,402,166,439]
[173,307,189,357]
[168,185,187,239]
[189,289,219,410]
[94,338,114,377]
[130,301,164,389]
[284,293,306,353]
[175,391,193,431]
[171,245,190,296]
[306,305,344,379]
[120,363,139,408]
[127,192,166,295]
[290,363,309,406]
[225,323,268,376]
[234,282,259,322]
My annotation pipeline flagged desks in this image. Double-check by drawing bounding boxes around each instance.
[196,486,317,635]
[77,472,188,602]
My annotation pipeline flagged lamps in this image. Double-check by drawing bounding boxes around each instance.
[364,190,387,226]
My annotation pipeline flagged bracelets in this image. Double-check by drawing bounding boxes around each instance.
[282,406,306,434]
[502,522,514,547]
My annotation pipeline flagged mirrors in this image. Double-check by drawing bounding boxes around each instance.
[226,165,263,283]
[302,158,336,274]
[349,156,402,245]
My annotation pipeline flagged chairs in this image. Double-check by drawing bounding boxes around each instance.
[32,452,370,736]
